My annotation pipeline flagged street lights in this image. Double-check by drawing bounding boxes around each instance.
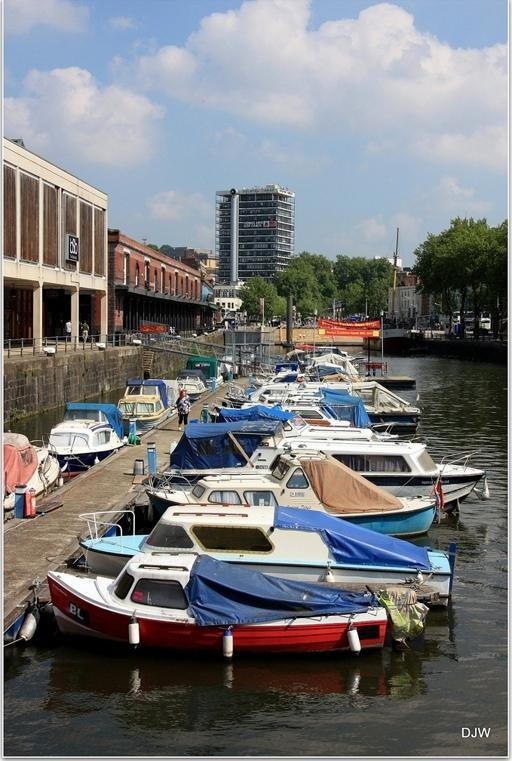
[378,309,384,377]
[311,308,318,362]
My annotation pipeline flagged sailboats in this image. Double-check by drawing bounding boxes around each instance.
[362,226,422,351]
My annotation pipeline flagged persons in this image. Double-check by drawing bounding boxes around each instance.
[176,390,191,430]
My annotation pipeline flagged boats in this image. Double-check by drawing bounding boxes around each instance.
[4,431,62,514]
[147,450,436,538]
[78,504,452,605]
[115,374,178,425]
[46,402,131,471]
[44,552,429,660]
